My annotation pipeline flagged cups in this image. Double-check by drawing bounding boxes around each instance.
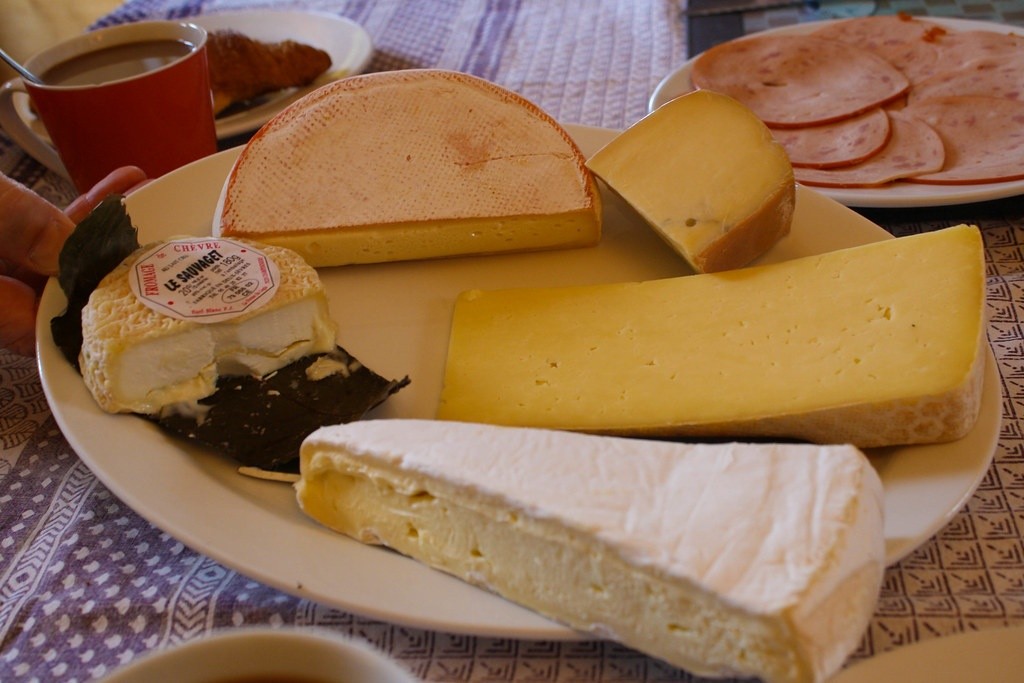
[0,21,220,197]
[96,626,420,683]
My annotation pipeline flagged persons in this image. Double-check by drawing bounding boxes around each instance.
[0,166,156,357]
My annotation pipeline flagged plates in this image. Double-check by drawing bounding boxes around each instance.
[35,124,1005,637]
[0,9,374,187]
[649,18,1024,208]
[832,625,1024,683]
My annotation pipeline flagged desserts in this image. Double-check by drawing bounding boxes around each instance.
[80,235,336,414]
[298,420,884,683]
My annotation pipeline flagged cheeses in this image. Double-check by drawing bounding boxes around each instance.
[585,90,796,273]
[211,68,602,268]
[440,223,986,445]
